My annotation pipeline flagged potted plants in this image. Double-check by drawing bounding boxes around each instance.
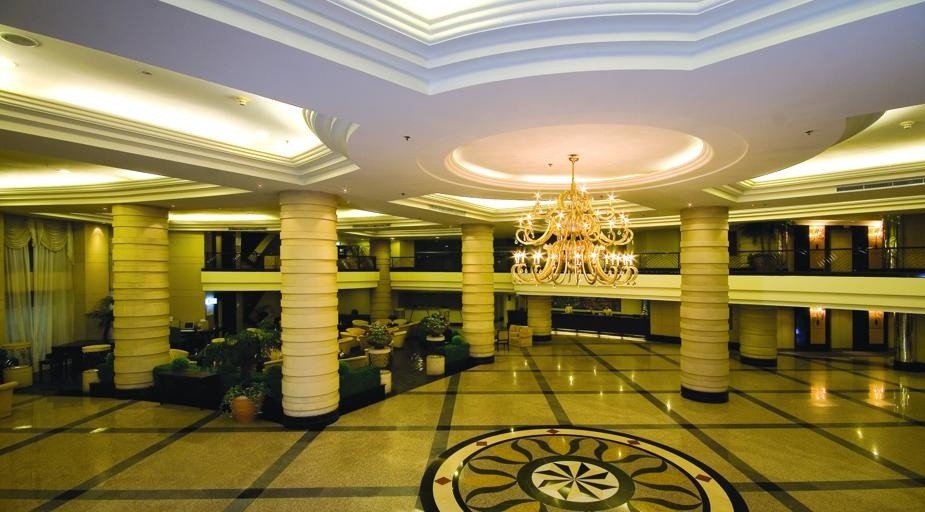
[218,382,273,425]
[426,311,449,338]
[364,324,394,368]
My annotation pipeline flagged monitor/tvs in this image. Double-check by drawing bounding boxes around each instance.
[184,322,194,329]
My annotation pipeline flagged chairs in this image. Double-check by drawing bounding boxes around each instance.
[494,325,533,352]
[338,318,422,359]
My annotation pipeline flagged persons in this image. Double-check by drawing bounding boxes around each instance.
[602,303,612,316]
[262,317,282,361]
[339,256,351,271]
[565,303,572,313]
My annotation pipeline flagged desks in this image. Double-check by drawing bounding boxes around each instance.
[156,372,222,410]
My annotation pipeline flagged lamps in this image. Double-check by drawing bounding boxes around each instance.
[510,152,640,287]
